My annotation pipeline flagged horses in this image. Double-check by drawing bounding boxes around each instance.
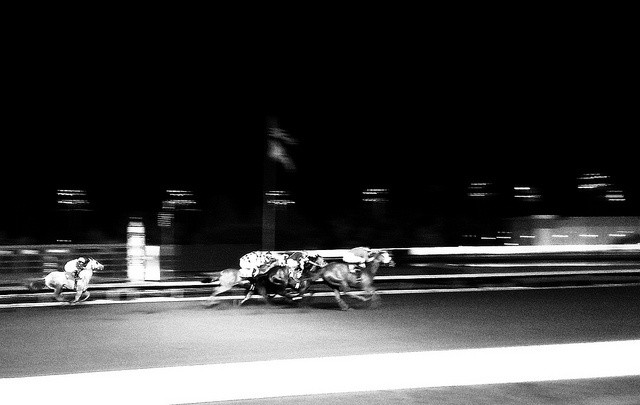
[303,250,396,312]
[29,257,105,303]
[203,255,329,309]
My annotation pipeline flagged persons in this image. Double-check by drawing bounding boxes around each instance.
[239,251,274,273]
[342,247,377,268]
[280,252,308,266]
[64,256,85,282]
